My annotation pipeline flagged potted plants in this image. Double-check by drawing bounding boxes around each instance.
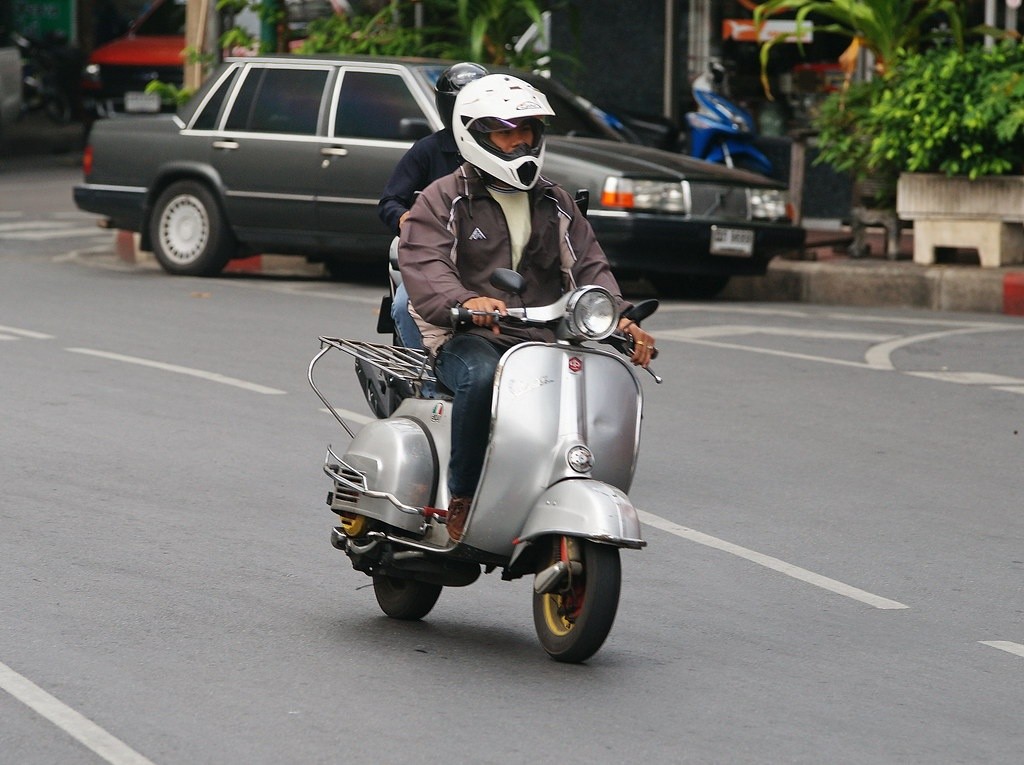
[809,34,1024,268]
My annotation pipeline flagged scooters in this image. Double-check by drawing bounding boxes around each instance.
[306,267,662,665]
[614,54,789,183]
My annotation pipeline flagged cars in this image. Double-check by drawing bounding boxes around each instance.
[72,56,809,302]
[76,1,356,115]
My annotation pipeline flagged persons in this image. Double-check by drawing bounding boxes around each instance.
[377,63,489,399]
[397,73,656,542]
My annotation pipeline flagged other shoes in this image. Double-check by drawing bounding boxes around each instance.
[447,494,472,543]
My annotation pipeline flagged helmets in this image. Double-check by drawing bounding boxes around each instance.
[433,62,491,134]
[452,74,557,192]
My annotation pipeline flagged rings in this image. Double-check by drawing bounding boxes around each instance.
[646,345,653,350]
[636,341,644,346]
